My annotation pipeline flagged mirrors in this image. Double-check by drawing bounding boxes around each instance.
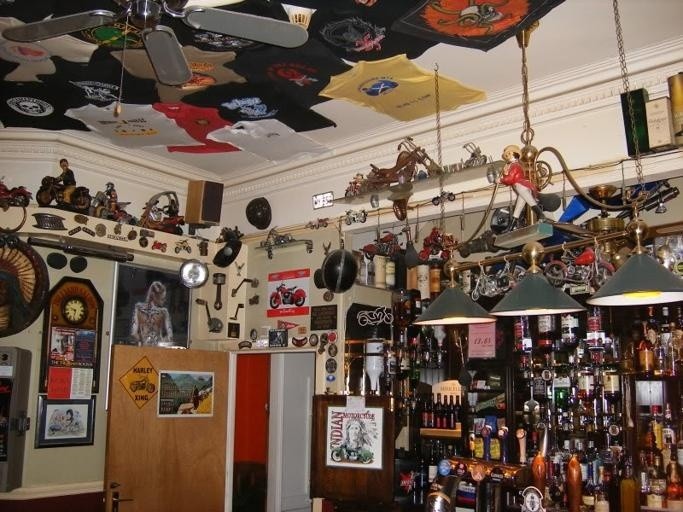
[341,299,395,398]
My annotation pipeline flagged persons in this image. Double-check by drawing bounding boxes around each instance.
[130,281,174,347]
[56,158,76,204]
[494,145,545,231]
[345,419,366,449]
[66,409,74,427]
[51,334,69,355]
[193,384,200,410]
[106,182,118,212]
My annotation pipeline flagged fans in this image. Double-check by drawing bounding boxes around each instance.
[0,0,310,87]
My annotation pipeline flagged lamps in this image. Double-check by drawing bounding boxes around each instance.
[408,0,683,324]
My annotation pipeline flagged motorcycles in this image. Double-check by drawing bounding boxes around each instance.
[270,283,305,309]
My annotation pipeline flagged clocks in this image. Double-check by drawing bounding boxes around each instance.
[61,294,89,324]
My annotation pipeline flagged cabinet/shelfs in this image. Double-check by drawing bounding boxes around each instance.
[392,223,683,489]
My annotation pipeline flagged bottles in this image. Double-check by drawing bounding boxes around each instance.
[637,403,682,512]
[412,437,458,493]
[396,324,412,377]
[421,392,461,429]
[511,304,625,512]
[620,454,637,512]
[637,305,682,376]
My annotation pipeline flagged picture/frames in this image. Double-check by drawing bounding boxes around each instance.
[34,394,96,449]
